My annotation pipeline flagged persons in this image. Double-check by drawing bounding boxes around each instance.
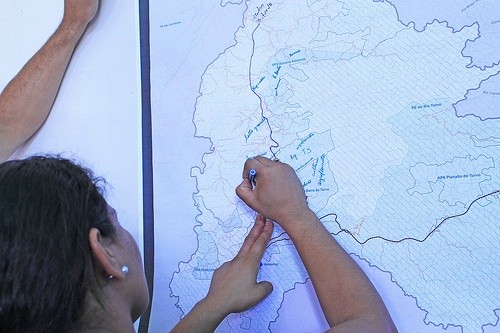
[0,154,399,332]
[0,0,100,162]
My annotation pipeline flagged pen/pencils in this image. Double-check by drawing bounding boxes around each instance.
[248,168,256,183]
